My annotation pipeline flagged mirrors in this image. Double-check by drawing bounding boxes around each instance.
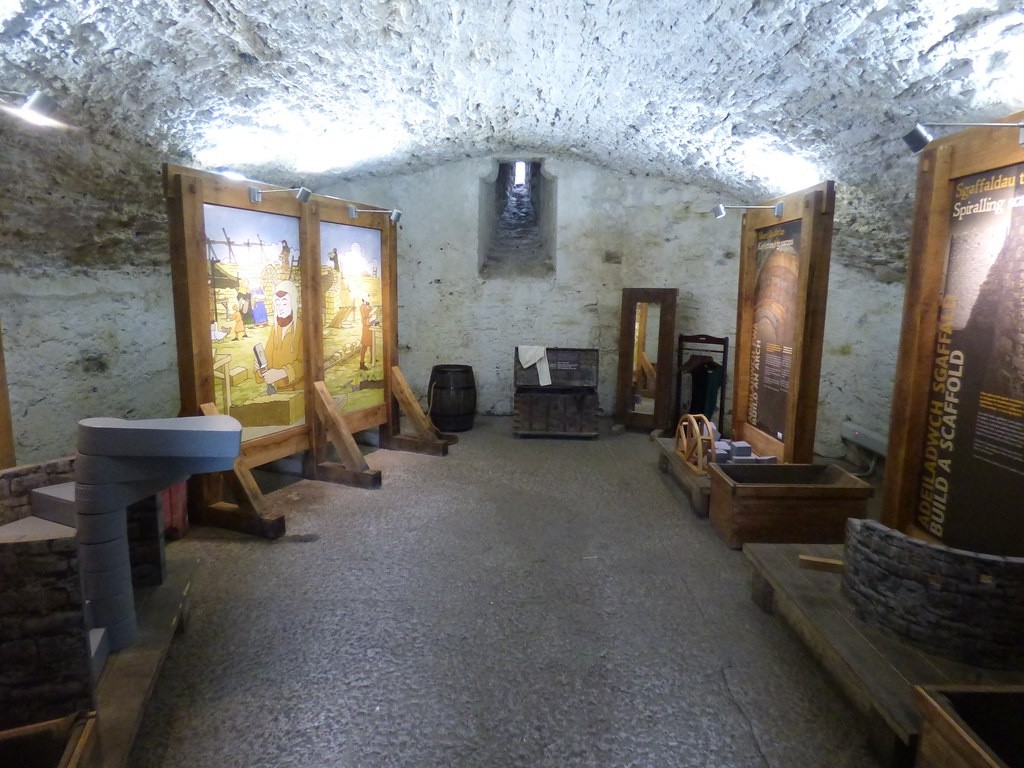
[614,287,678,429]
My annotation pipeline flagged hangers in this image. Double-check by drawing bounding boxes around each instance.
[683,345,715,372]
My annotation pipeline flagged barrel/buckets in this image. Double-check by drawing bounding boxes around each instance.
[428,365,476,432]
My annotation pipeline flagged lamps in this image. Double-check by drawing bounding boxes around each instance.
[711,200,785,218]
[348,203,403,224]
[900,120,1024,154]
[248,185,312,205]
